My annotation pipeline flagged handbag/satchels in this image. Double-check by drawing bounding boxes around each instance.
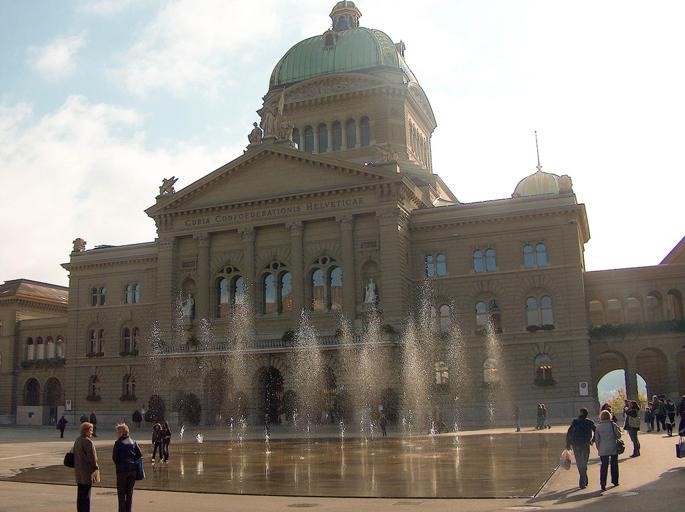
[629,416,640,429]
[64,453,74,468]
[135,457,145,480]
[616,440,625,454]
[676,436,685,458]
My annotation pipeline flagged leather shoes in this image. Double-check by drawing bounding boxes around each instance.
[601,486,606,492]
[612,481,619,486]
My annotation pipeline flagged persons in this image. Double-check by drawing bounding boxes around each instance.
[151,423,163,462]
[364,277,377,302]
[247,122,263,144]
[58,415,68,438]
[112,423,143,512]
[90,410,97,437]
[80,412,88,424]
[535,393,685,492]
[157,422,171,463]
[180,293,194,316]
[73,422,99,512]
[379,414,388,437]
[512,406,520,432]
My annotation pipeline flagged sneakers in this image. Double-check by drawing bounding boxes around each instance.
[152,459,155,462]
